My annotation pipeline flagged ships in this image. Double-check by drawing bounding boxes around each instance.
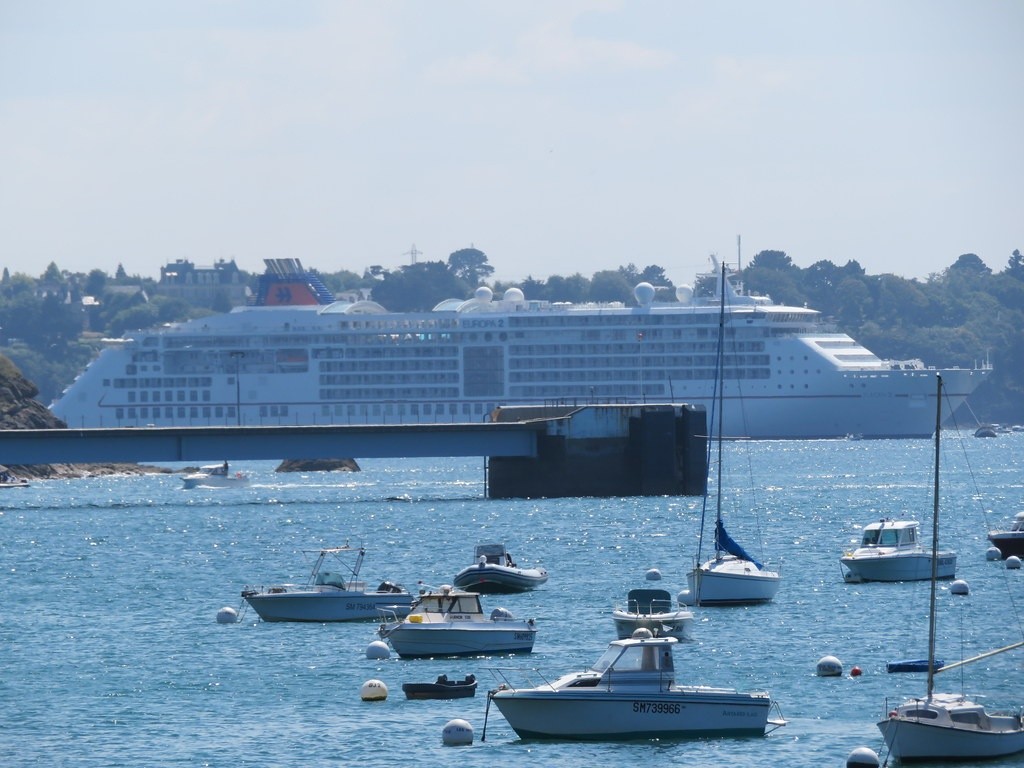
[45,257,995,441]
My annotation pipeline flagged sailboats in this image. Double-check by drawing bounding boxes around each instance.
[686,258,784,606]
[877,373,1024,763]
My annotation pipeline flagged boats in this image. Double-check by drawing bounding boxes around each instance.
[401,673,479,701]
[478,627,792,742]
[241,538,421,624]
[887,658,946,674]
[181,457,250,488]
[378,583,539,659]
[453,539,550,596]
[986,510,1024,559]
[614,586,696,644]
[839,515,957,584]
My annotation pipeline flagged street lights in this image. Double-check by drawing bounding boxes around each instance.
[231,351,245,426]
